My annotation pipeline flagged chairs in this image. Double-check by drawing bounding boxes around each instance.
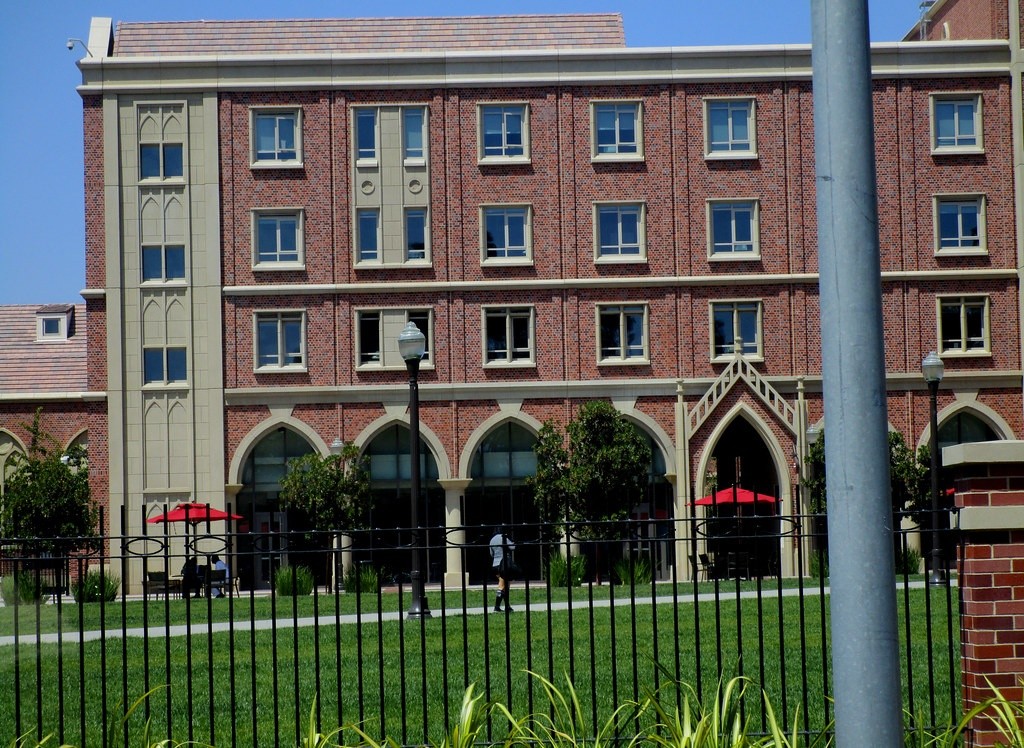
[685,549,777,581]
[138,564,240,600]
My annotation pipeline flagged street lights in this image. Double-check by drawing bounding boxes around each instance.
[922,350,949,586]
[398,321,433,619]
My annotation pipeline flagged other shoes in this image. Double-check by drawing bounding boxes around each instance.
[216,593,225,598]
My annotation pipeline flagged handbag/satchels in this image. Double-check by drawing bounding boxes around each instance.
[499,557,518,581]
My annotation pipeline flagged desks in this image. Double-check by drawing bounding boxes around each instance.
[172,573,205,599]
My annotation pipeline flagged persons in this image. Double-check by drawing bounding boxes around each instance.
[488,525,516,613]
[206,554,230,599]
[181,558,201,599]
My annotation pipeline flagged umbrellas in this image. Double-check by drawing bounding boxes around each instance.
[145,501,244,566]
[685,486,783,579]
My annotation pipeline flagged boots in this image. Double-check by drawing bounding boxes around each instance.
[494,596,503,611]
[510,607,513,611]
